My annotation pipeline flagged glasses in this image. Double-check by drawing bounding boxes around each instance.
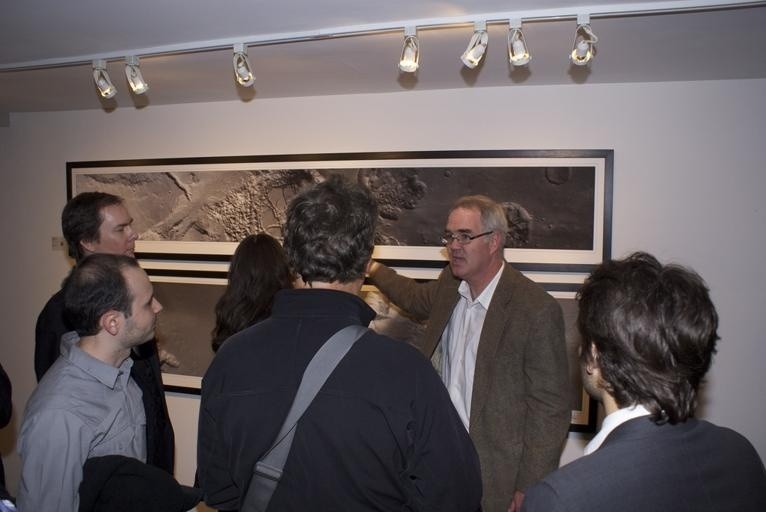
[568,13,599,66]
[507,17,531,67]
[397,25,420,74]
[92,60,117,100]
[232,43,255,87]
[124,55,149,96]
[461,20,487,71]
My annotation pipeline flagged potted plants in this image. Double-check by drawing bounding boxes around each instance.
[65,149,614,272]
[132,269,599,434]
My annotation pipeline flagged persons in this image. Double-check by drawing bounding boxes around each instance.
[209,234,297,357]
[195,176,485,511]
[33,190,175,476]
[518,251,766,511]
[363,194,573,512]
[16,253,164,512]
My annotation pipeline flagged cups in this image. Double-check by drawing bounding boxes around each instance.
[440,230,492,244]
